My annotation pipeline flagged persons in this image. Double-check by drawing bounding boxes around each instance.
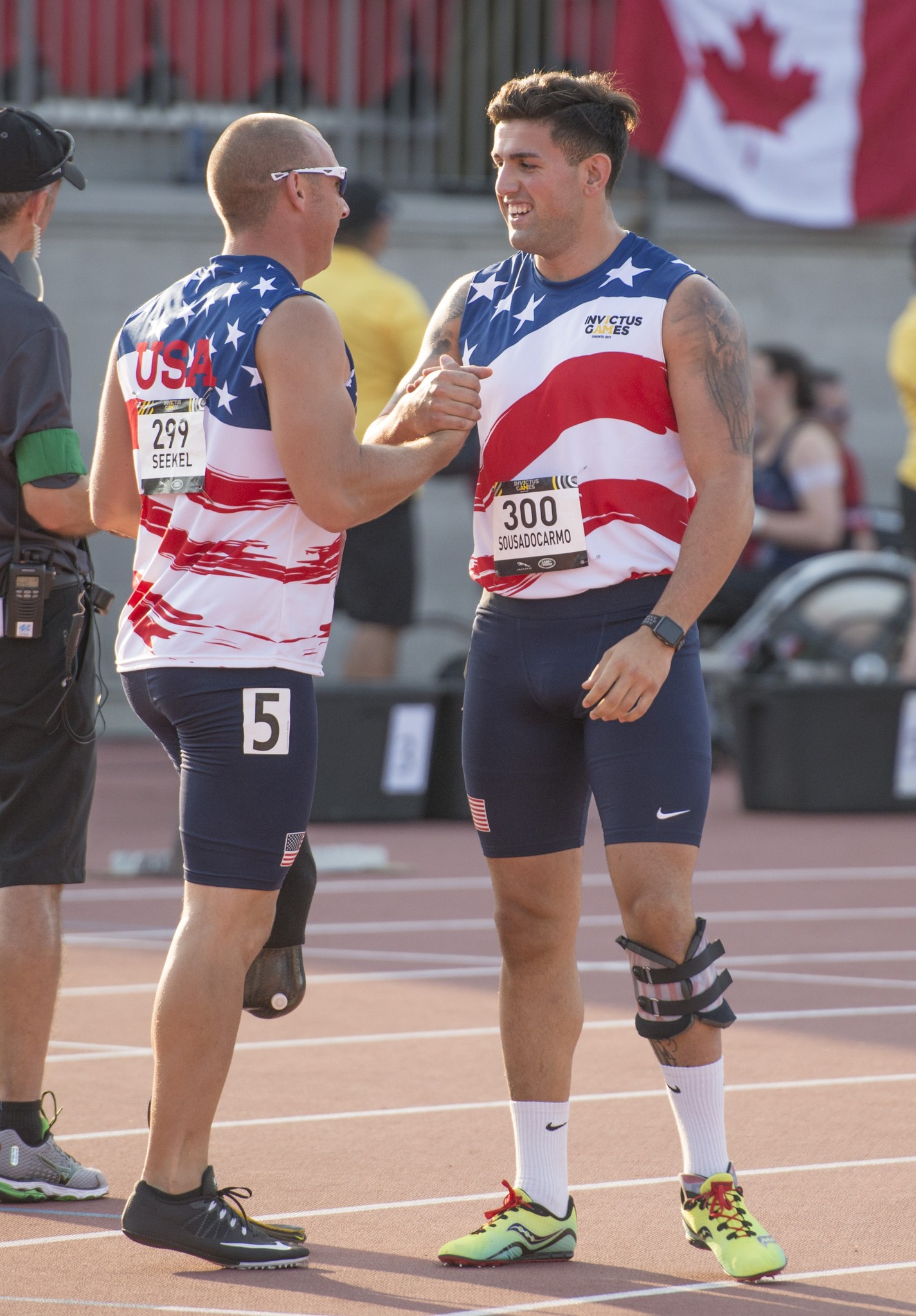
[699,234,916,803]
[86,71,790,1289]
[300,177,433,683]
[0,108,115,1208]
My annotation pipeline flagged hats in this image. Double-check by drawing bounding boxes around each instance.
[1,105,86,194]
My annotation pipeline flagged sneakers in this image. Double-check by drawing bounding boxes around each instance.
[678,1160,789,1286]
[436,1179,579,1267]
[1,1090,109,1201]
[122,1168,311,1268]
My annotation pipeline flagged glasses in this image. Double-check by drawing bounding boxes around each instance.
[272,165,347,198]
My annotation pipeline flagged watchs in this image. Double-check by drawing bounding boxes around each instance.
[640,613,686,652]
